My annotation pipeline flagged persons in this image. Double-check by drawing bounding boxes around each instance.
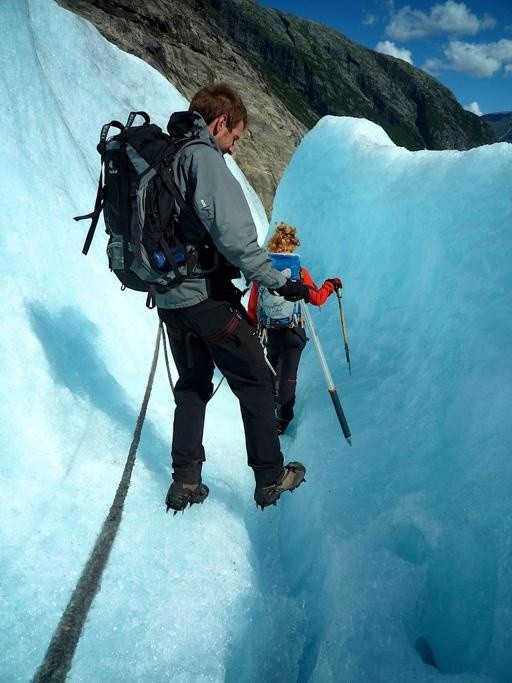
[94,83,307,510]
[244,222,344,437]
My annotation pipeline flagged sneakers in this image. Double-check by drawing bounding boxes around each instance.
[165,479,209,516]
[254,461,306,511]
[275,417,289,435]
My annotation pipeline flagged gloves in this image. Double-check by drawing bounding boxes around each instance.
[276,279,310,304]
[328,278,342,293]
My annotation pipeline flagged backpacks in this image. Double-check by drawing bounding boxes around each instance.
[256,252,301,329]
[97,111,219,295]
